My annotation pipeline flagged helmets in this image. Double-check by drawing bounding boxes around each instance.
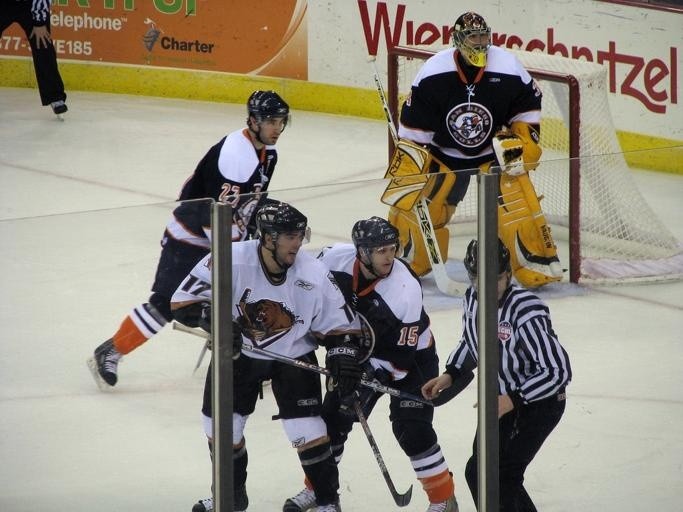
[247,90,290,117]
[256,202,308,230]
[351,216,400,248]
[451,11,492,68]
[463,237,511,276]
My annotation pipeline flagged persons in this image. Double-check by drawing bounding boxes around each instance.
[381,13,570,288]
[421,238,571,512]
[170,200,359,512]
[0,1,69,122]
[285,216,459,512]
[86,90,292,393]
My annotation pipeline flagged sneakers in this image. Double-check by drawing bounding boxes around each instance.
[306,498,342,512]
[282,486,318,512]
[51,99,68,114]
[425,494,460,512]
[191,483,249,512]
[94,337,123,386]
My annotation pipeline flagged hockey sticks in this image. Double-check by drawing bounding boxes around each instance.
[367,55,469,298]
[352,391,413,506]
[172,322,475,409]
